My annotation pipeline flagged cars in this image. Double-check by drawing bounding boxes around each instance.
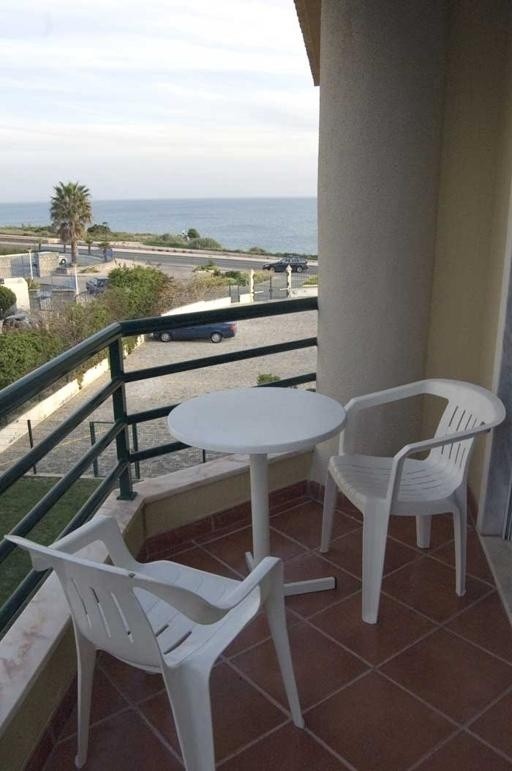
[262,256,309,274]
[151,321,238,344]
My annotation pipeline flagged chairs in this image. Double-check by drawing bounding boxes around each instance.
[1,514,306,771]
[318,376,508,628]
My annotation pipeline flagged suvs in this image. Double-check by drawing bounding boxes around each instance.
[33,251,68,267]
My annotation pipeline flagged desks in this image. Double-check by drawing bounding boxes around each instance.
[167,384,347,601]
